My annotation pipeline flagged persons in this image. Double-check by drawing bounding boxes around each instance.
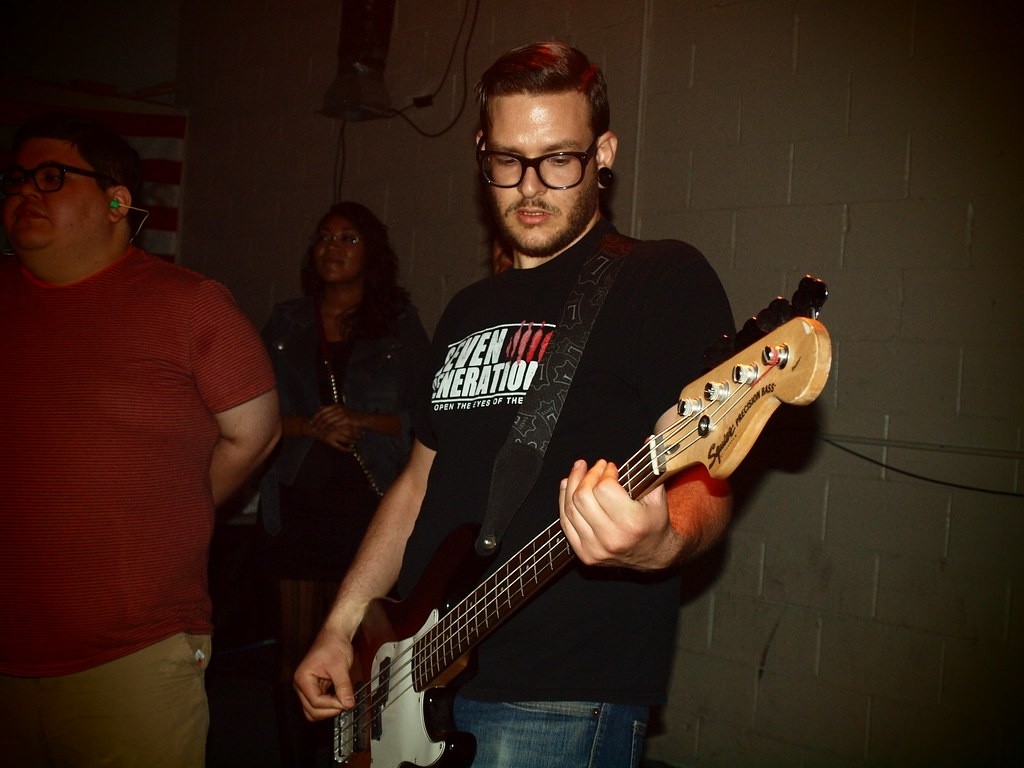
[289,198,429,767]
[0,114,278,768]
[289,38,737,768]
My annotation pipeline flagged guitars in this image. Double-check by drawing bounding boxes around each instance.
[332,275,834,767]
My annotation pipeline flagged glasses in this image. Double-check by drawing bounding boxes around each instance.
[316,232,365,247]
[476,136,598,189]
[0,161,96,194]
